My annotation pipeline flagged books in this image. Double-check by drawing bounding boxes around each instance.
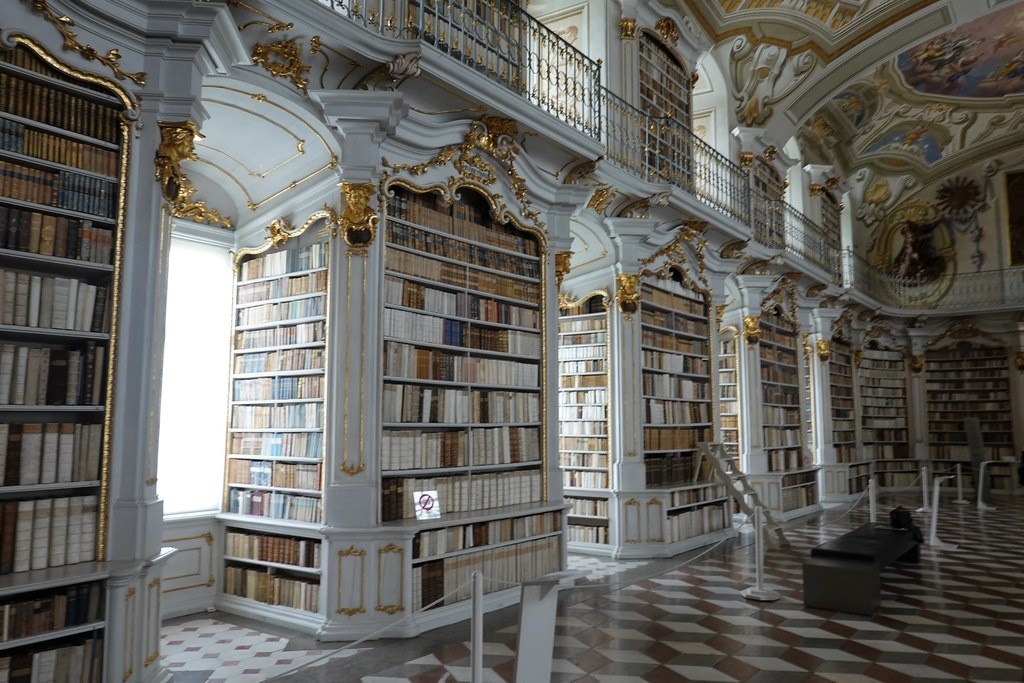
[641,286,713,487]
[0,45,120,682]
[667,486,730,544]
[829,341,1013,494]
[759,314,816,509]
[384,195,542,519]
[640,36,687,181]
[409,0,520,87]
[411,512,563,614]
[755,163,841,245]
[557,295,609,544]
[718,341,739,513]
[224,240,329,613]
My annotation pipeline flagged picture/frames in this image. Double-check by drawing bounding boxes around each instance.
[1002,168,1024,269]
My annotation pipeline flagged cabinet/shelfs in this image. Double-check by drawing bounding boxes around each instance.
[0,60,119,681]
[216,0,1024,640]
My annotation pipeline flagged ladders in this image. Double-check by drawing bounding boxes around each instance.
[698,441,790,552]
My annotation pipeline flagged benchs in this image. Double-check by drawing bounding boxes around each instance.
[801,522,923,611]
